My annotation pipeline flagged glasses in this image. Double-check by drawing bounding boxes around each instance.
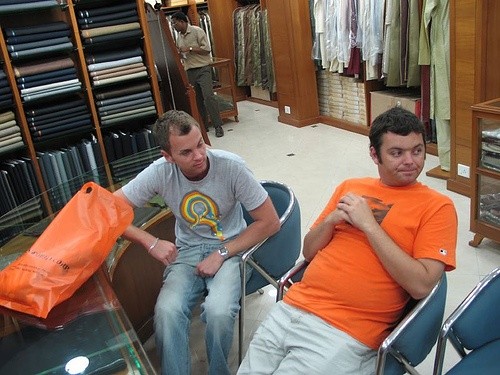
[171,21,181,26]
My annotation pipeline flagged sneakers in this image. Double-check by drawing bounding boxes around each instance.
[204,126,209,132]
[215,126,224,137]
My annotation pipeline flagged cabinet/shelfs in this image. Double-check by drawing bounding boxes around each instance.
[0,0,176,248]
[467,98,500,247]
[199,58,239,125]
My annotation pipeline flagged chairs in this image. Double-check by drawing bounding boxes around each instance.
[239,180,300,366]
[433,268,500,375]
[277,258,448,375]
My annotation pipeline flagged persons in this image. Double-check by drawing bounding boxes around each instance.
[171,12,224,137]
[236,107,457,375]
[112,110,281,375]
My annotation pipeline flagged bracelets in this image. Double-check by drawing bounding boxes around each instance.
[148,238,159,253]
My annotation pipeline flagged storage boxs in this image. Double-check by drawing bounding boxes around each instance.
[369,89,431,142]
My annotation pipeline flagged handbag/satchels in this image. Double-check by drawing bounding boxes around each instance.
[0,182,134,319]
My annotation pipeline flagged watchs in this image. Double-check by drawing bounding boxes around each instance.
[217,246,229,259]
[189,46,192,52]
